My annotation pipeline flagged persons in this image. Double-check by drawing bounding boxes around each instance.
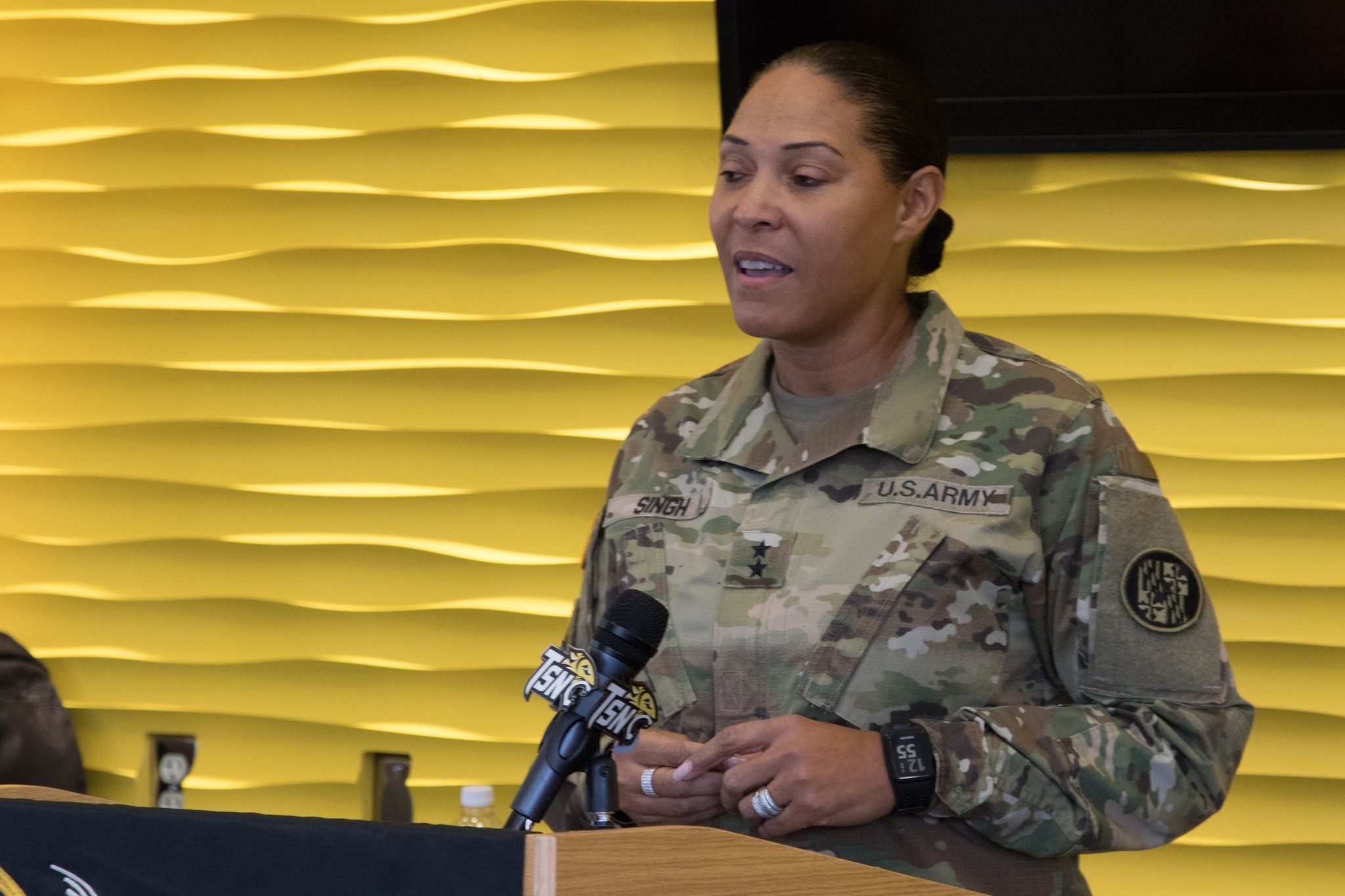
[541,41,1254,895]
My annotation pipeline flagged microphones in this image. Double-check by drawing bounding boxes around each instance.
[503,588,669,831]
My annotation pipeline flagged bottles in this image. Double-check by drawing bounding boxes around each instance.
[456,785,496,829]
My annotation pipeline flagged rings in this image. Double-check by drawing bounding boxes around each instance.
[751,784,782,819]
[639,765,660,797]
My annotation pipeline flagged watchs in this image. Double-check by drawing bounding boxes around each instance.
[875,718,937,818]
